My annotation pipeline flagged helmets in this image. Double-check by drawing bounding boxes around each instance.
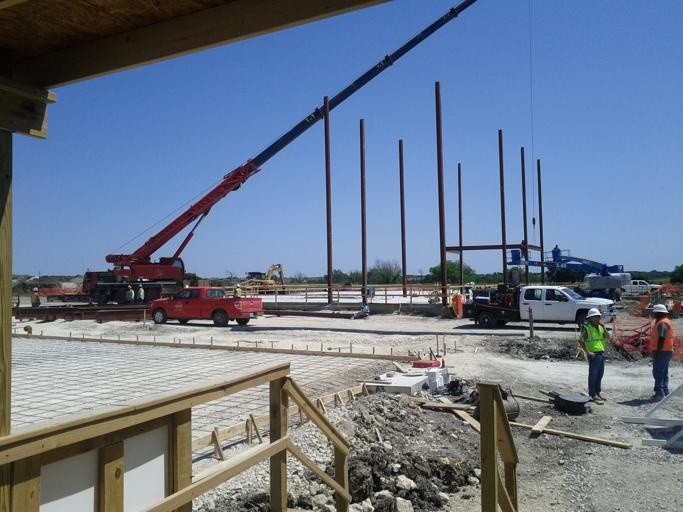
[652,303,669,313]
[585,308,602,319]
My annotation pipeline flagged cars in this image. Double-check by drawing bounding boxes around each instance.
[621,279,662,293]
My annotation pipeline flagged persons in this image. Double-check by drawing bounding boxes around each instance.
[134,284,144,304]
[667,299,682,320]
[650,303,676,400]
[123,284,135,304]
[577,308,616,403]
[30,287,41,307]
[233,283,241,297]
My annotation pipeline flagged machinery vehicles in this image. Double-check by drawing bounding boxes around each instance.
[507,246,630,292]
[82,1,478,304]
[258,264,286,295]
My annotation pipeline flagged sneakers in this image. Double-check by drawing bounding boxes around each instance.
[648,393,669,403]
[590,393,607,401]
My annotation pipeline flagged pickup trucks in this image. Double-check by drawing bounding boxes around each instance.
[150,286,263,328]
[463,285,617,328]
